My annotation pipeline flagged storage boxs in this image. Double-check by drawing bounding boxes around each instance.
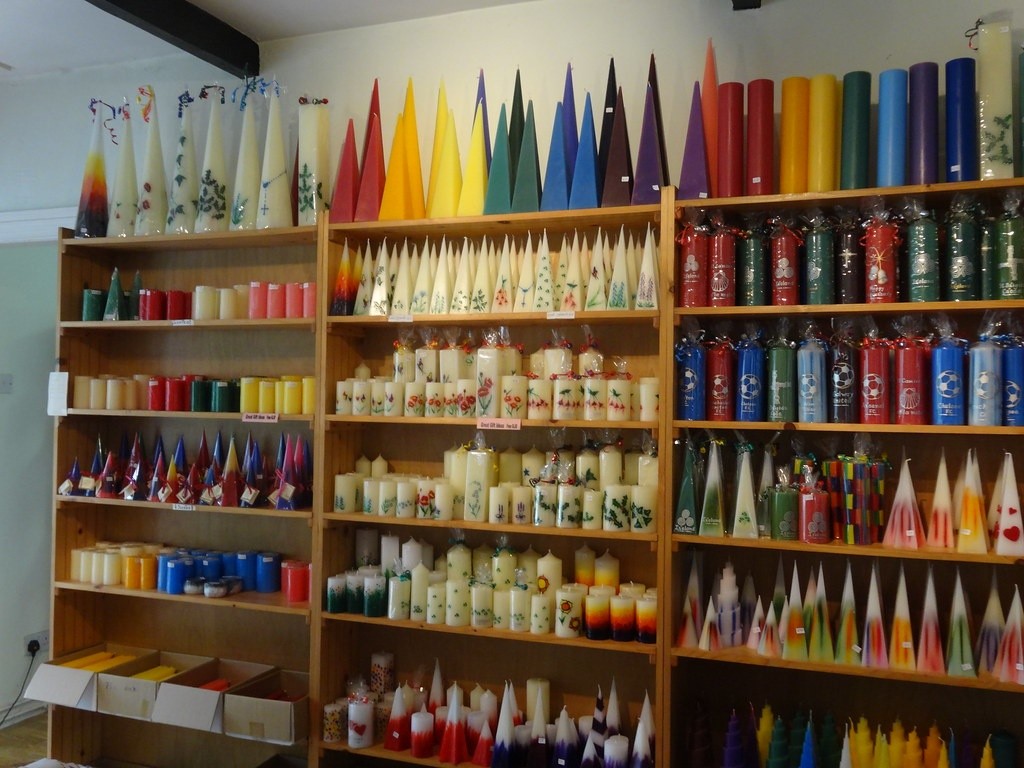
[224,668,311,744]
[23,641,159,711]
[98,650,217,720]
[150,658,279,734]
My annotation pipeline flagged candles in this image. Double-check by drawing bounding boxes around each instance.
[57,21,1023,768]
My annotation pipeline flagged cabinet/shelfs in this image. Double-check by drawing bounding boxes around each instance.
[49,208,323,768]
[663,176,1024,767]
[314,184,667,767]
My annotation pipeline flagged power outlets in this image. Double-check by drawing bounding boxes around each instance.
[24,632,41,657]
[40,630,48,651]
